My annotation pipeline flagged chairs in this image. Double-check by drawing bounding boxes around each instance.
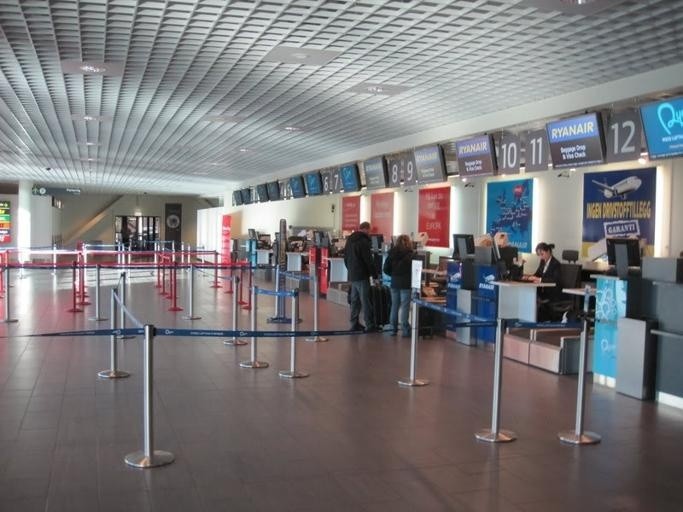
[546,250,582,319]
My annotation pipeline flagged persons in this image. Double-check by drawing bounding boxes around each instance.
[330,237,341,254]
[520,242,564,323]
[344,221,381,332]
[382,233,419,338]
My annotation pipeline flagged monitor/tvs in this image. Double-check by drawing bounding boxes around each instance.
[363,155,388,190]
[246,229,641,289]
[413,143,447,184]
[455,134,497,179]
[229,164,361,206]
[545,111,606,170]
[639,96,683,161]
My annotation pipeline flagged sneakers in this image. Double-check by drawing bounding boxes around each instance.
[350,324,365,331]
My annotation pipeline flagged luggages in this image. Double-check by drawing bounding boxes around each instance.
[418,305,443,340]
[367,285,392,330]
